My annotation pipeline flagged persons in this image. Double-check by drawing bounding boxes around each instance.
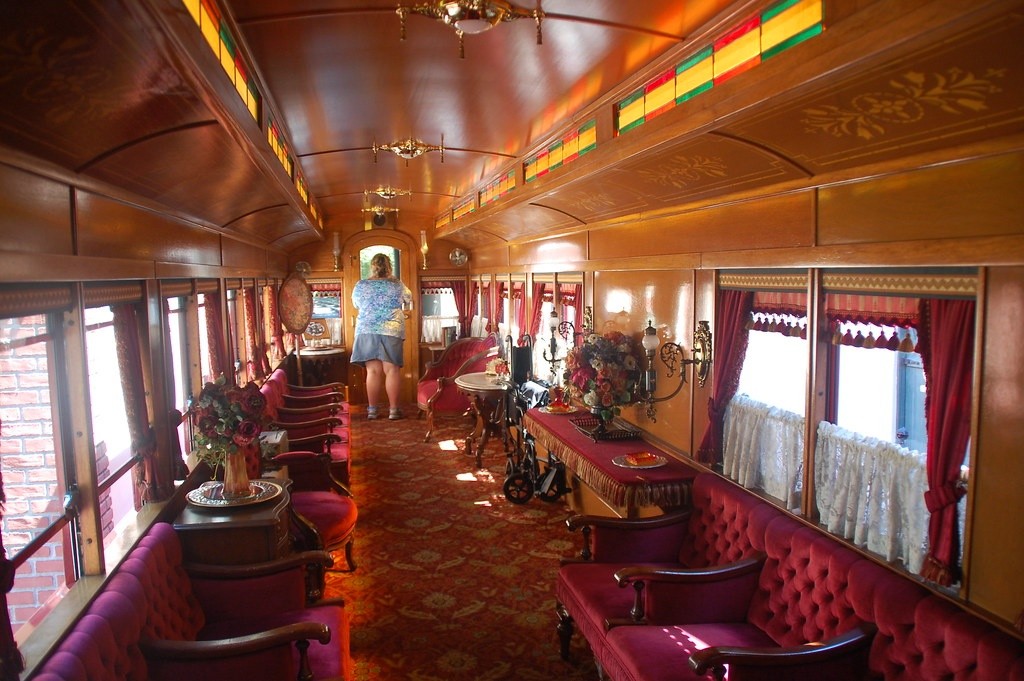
[350,254,414,420]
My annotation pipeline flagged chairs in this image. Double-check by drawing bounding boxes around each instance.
[414,335,500,441]
[265,370,351,427]
[255,384,352,493]
[26,522,352,681]
[547,468,1024,681]
[261,450,360,571]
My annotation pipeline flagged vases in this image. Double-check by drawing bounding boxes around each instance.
[589,404,609,434]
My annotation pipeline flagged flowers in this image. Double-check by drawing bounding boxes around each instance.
[560,327,641,428]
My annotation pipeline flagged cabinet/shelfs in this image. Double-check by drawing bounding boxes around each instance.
[172,476,296,562]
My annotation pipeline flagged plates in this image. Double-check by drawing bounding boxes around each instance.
[539,404,579,415]
[612,453,668,469]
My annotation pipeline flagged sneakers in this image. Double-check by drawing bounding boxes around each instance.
[368,406,381,418]
[389,408,405,420]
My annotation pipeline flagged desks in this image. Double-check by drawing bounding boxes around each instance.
[292,345,345,387]
[521,402,699,521]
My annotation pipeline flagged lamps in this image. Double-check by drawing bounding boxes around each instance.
[542,305,592,371]
[419,229,429,270]
[631,318,712,422]
[332,230,341,271]
